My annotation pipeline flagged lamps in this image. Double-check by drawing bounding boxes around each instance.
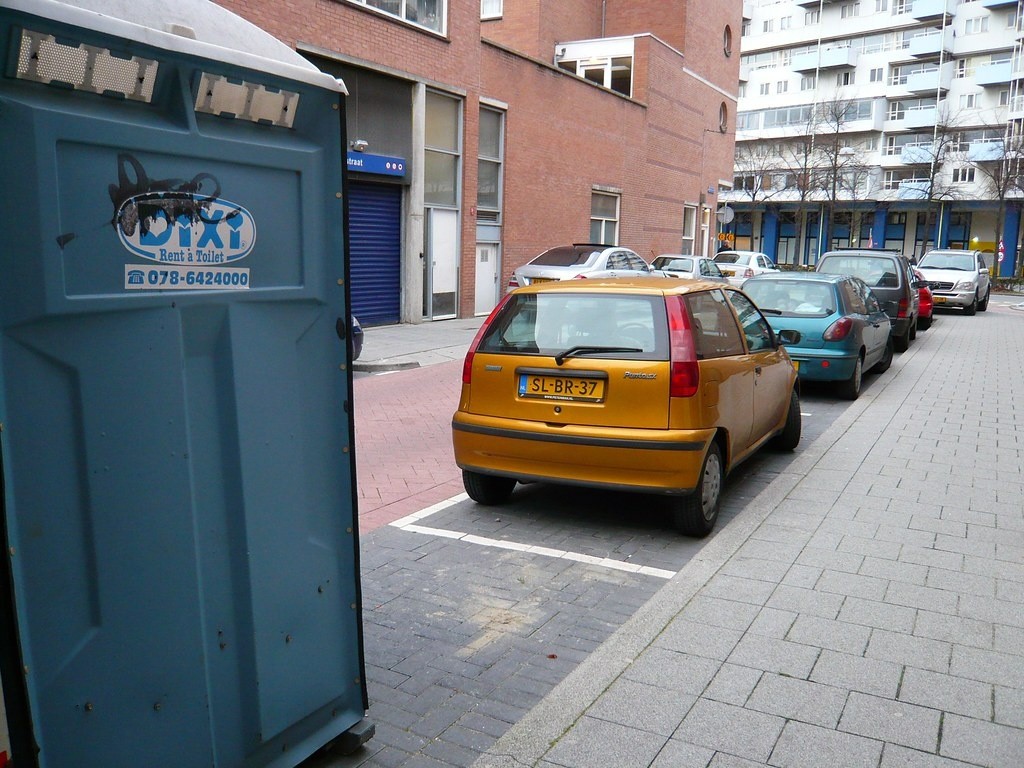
[349,140,368,152]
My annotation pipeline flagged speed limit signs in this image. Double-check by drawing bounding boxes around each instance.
[997,252,1005,262]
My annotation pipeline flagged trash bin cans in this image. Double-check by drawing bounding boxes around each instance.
[0,0,377,768]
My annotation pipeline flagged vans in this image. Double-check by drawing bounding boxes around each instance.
[815,248,920,354]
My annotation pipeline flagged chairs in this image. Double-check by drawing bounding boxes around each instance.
[769,291,791,305]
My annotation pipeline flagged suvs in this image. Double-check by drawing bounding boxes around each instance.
[913,250,991,316]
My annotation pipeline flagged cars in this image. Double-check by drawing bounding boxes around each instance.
[710,251,781,288]
[452,284,802,538]
[913,270,933,330]
[506,244,664,320]
[647,255,731,287]
[714,272,894,400]
